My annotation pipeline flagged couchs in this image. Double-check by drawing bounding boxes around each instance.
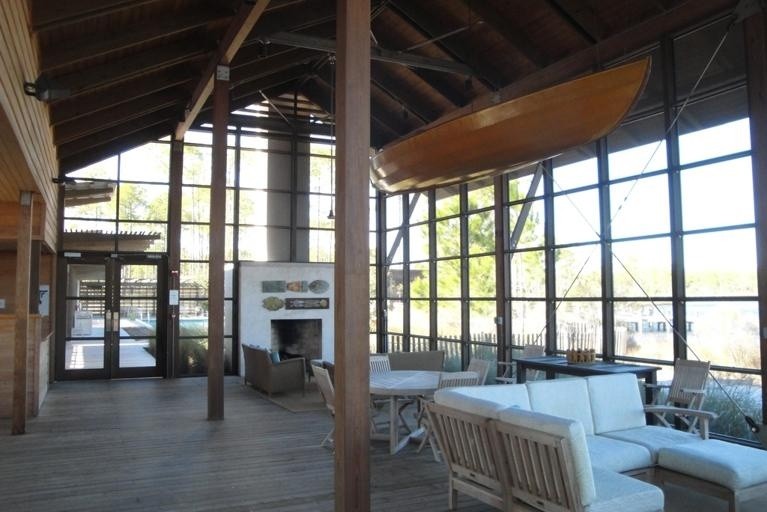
[422,372,766,512]
[241,343,306,399]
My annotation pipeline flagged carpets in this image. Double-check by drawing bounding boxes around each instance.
[239,376,329,413]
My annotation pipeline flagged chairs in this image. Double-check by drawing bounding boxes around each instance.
[643,359,711,434]
[369,354,414,435]
[467,358,491,385]
[416,373,481,463]
[312,365,377,455]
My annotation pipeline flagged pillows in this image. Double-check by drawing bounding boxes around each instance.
[249,344,280,366]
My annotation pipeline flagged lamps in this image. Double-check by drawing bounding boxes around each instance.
[327,54,338,220]
[53,173,76,190]
[24,69,68,102]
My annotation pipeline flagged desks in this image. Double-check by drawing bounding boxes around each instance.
[511,355,662,425]
